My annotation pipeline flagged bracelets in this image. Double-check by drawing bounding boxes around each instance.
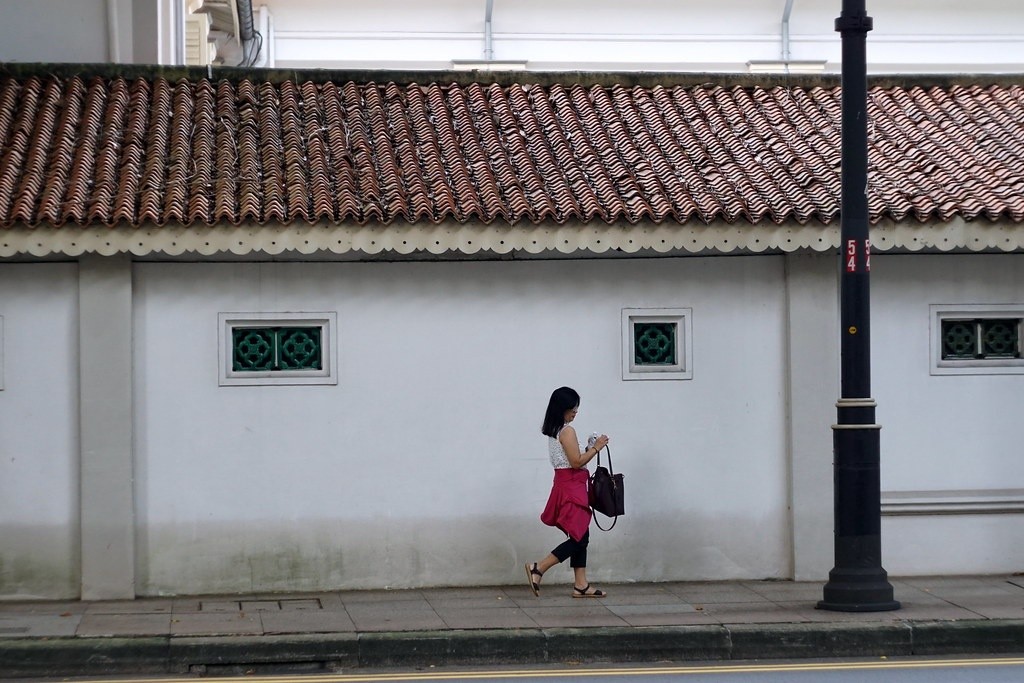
[592,446,598,453]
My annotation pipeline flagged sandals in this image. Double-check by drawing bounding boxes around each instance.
[573,583,607,598]
[525,563,542,597]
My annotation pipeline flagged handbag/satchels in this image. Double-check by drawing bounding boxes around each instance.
[588,444,625,531]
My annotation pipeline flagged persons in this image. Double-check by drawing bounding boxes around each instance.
[525,387,610,599]
